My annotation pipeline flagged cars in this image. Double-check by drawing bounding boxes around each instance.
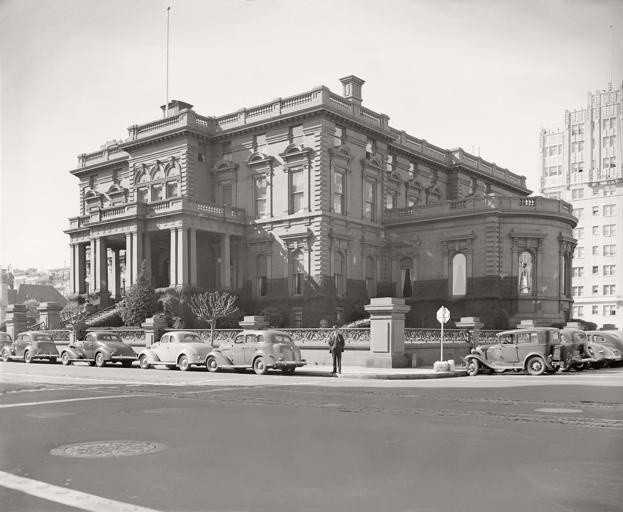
[59,331,138,368]
[205,329,307,376]
[1,331,59,364]
[0,331,13,359]
[138,331,214,371]
[463,328,623,376]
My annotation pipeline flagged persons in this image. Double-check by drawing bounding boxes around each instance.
[327,324,345,374]
[519,263,530,293]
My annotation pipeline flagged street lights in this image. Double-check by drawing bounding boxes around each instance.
[85,277,89,295]
